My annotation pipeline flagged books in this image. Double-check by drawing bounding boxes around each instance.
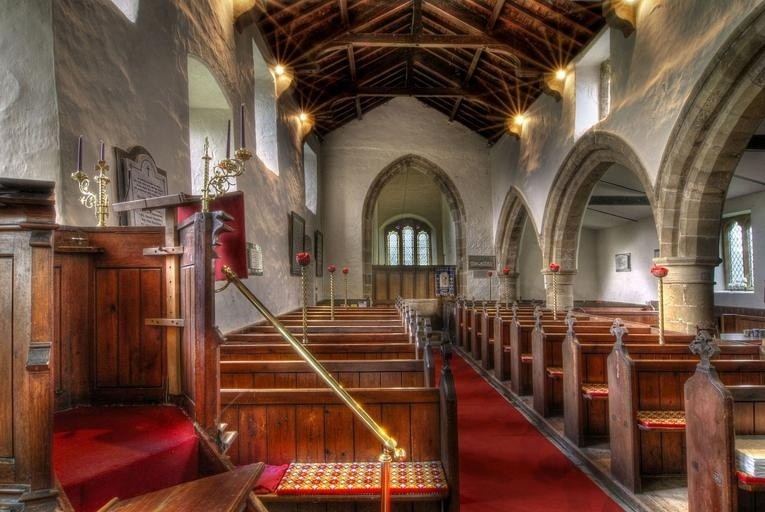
[734,434,764,477]
